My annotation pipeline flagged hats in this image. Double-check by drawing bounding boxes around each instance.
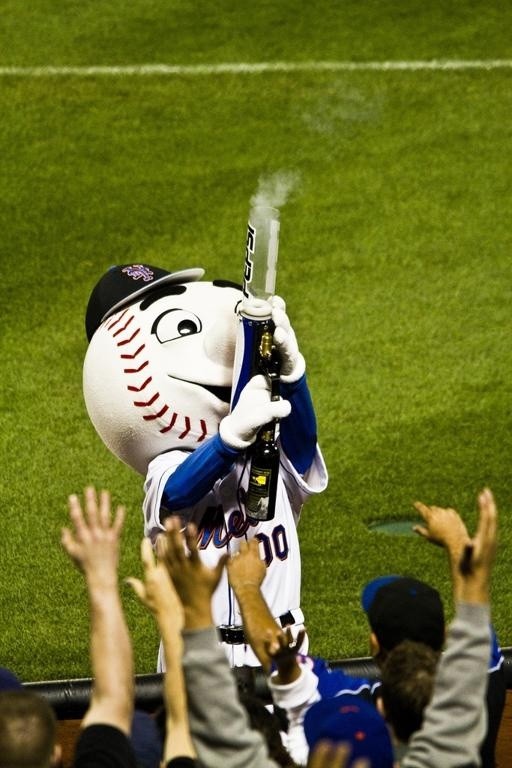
[360,573,442,655]
[303,694,393,768]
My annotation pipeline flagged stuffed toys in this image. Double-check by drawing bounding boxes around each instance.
[79,260,331,677]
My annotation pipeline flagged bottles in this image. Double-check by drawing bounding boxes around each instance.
[246,424,281,522]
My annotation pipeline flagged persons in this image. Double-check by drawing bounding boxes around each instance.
[1,479,512,767]
